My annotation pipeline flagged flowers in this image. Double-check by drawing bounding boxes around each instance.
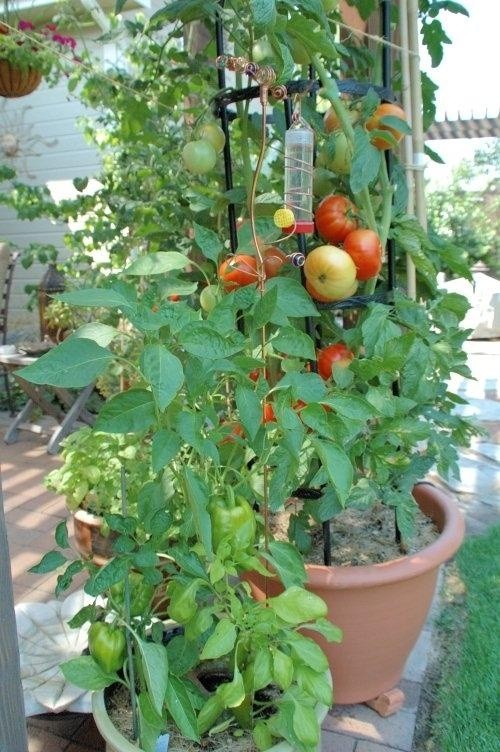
[0,19,77,69]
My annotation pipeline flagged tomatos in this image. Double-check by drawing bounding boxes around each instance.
[149,102,404,448]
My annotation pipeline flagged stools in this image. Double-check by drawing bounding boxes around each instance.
[1,342,106,459]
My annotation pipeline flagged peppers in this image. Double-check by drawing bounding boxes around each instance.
[88,611,128,673]
[207,485,255,566]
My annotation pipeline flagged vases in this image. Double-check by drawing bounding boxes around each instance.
[0,58,43,99]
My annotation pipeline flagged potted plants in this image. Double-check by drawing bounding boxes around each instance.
[80,475,332,752]
[67,332,197,609]
[234,297,475,711]
